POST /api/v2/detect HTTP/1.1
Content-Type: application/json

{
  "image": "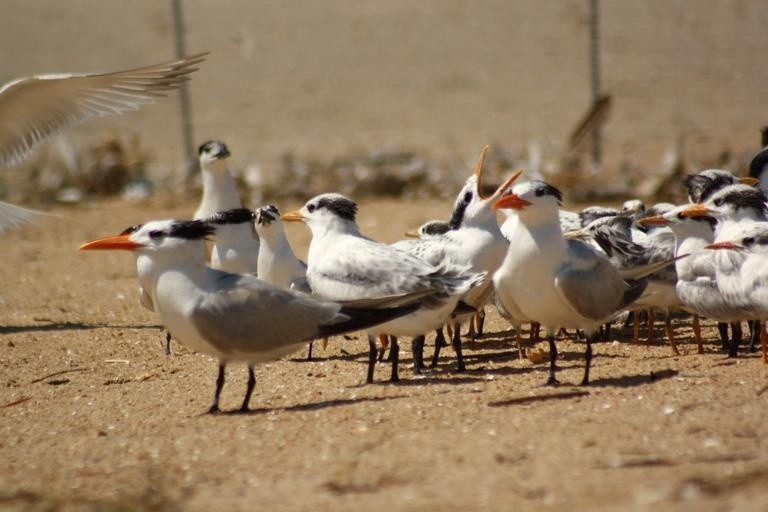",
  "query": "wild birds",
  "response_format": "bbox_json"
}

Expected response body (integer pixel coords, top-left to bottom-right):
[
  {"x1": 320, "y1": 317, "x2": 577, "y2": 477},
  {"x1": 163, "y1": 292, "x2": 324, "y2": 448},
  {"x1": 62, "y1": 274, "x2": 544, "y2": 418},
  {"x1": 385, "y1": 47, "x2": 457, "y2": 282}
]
[
  {"x1": 78, "y1": 220, "x2": 349, "y2": 416},
  {"x1": 196, "y1": 139, "x2": 768, "y2": 395},
  {"x1": 0, "y1": 52, "x2": 211, "y2": 236}
]
